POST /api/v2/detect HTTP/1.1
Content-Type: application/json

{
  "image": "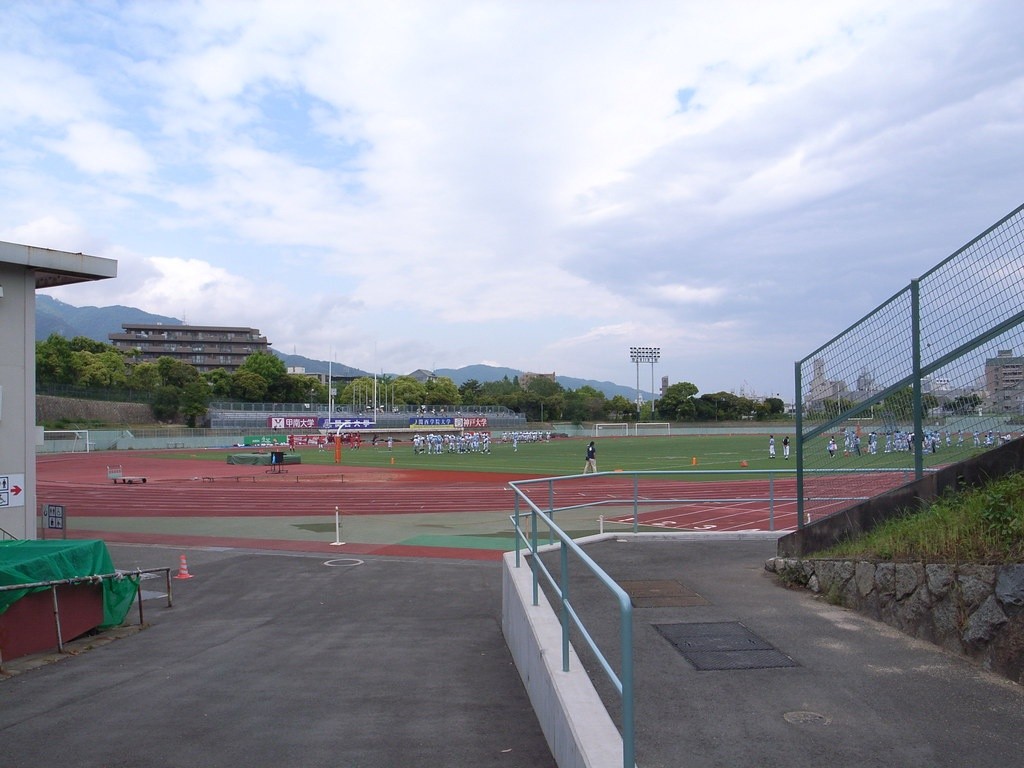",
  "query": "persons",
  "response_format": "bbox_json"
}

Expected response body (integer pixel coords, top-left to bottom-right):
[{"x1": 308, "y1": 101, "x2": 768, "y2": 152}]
[
  {"x1": 356, "y1": 405, "x2": 504, "y2": 419},
  {"x1": 289, "y1": 431, "x2": 393, "y2": 452},
  {"x1": 768, "y1": 435, "x2": 776, "y2": 459},
  {"x1": 413, "y1": 433, "x2": 491, "y2": 455},
  {"x1": 782, "y1": 436, "x2": 791, "y2": 459},
  {"x1": 828, "y1": 426, "x2": 1012, "y2": 457},
  {"x1": 501, "y1": 431, "x2": 551, "y2": 452},
  {"x1": 583, "y1": 441, "x2": 597, "y2": 475}
]
